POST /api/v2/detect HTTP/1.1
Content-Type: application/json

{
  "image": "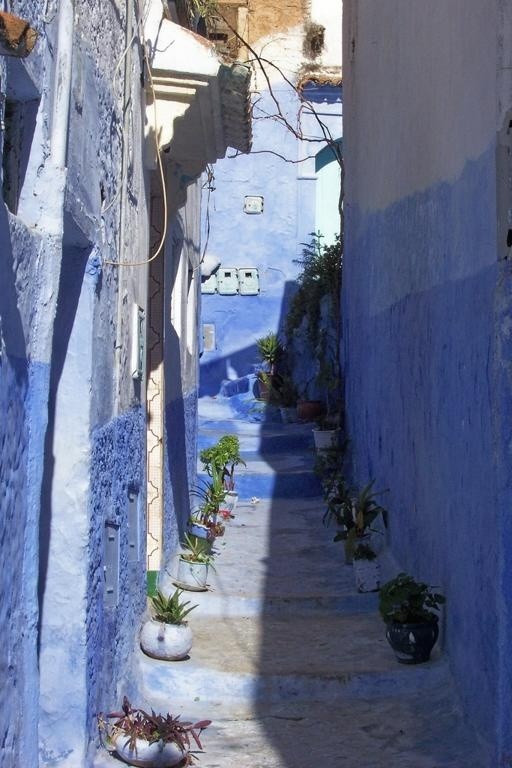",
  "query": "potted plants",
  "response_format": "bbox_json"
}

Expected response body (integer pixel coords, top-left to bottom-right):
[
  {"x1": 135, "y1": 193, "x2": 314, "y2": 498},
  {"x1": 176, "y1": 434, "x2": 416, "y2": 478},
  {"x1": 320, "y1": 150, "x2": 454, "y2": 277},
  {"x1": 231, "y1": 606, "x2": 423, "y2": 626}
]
[
  {"x1": 377, "y1": 571, "x2": 445, "y2": 665},
  {"x1": 186, "y1": 432, "x2": 249, "y2": 554},
  {"x1": 140, "y1": 583, "x2": 200, "y2": 661},
  {"x1": 173, "y1": 528, "x2": 212, "y2": 592},
  {"x1": 295, "y1": 356, "x2": 324, "y2": 421},
  {"x1": 96, "y1": 698, "x2": 212, "y2": 768},
  {"x1": 279, "y1": 341, "x2": 303, "y2": 423},
  {"x1": 256, "y1": 332, "x2": 284, "y2": 399},
  {"x1": 287, "y1": 296, "x2": 346, "y2": 455},
  {"x1": 317, "y1": 437, "x2": 392, "y2": 591}
]
